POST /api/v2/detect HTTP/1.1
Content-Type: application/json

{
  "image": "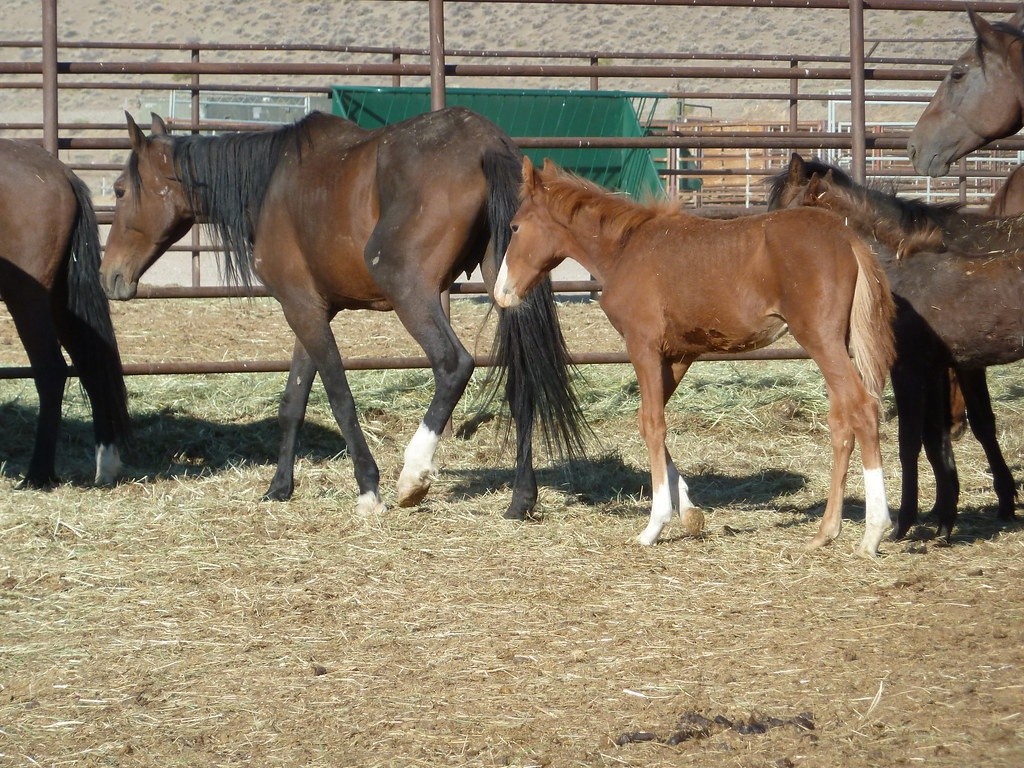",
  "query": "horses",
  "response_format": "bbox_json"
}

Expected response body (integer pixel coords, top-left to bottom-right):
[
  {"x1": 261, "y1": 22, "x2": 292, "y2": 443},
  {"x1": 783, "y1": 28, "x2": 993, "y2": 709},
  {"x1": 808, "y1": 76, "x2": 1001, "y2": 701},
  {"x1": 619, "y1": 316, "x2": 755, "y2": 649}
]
[
  {"x1": 493, "y1": 155, "x2": 899, "y2": 558},
  {"x1": 760, "y1": 4, "x2": 1024, "y2": 547},
  {"x1": 99, "y1": 110, "x2": 607, "y2": 522},
  {"x1": 0, "y1": 137, "x2": 136, "y2": 492}
]
